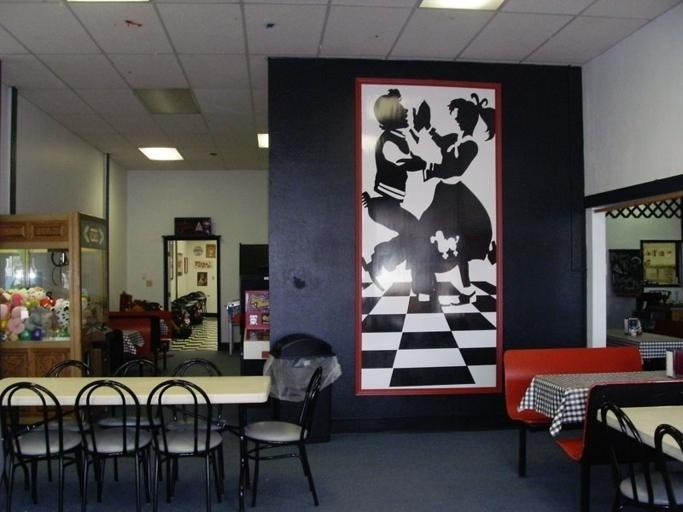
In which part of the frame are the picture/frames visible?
[355,78,503,396]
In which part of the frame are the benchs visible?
[504,347,642,478]
[553,382,682,511]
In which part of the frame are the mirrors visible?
[607,199,683,369]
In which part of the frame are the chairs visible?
[1,380,84,511]
[113,360,162,483]
[75,380,155,511]
[601,405,682,511]
[43,360,93,484]
[241,365,323,512]
[163,359,224,480]
[148,379,225,511]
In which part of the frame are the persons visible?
[361,89,439,303]
[395,92,497,308]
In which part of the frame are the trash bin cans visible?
[269,333,336,444]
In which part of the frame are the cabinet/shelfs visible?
[240,244,269,355]
[241,359,331,443]
[109,312,171,369]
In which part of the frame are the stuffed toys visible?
[0,286,104,343]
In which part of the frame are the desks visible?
[516,372,683,437]
[670,308,683,322]
[596,406,683,463]
[229,299,241,356]
[0,373,277,511]
[607,329,683,365]
